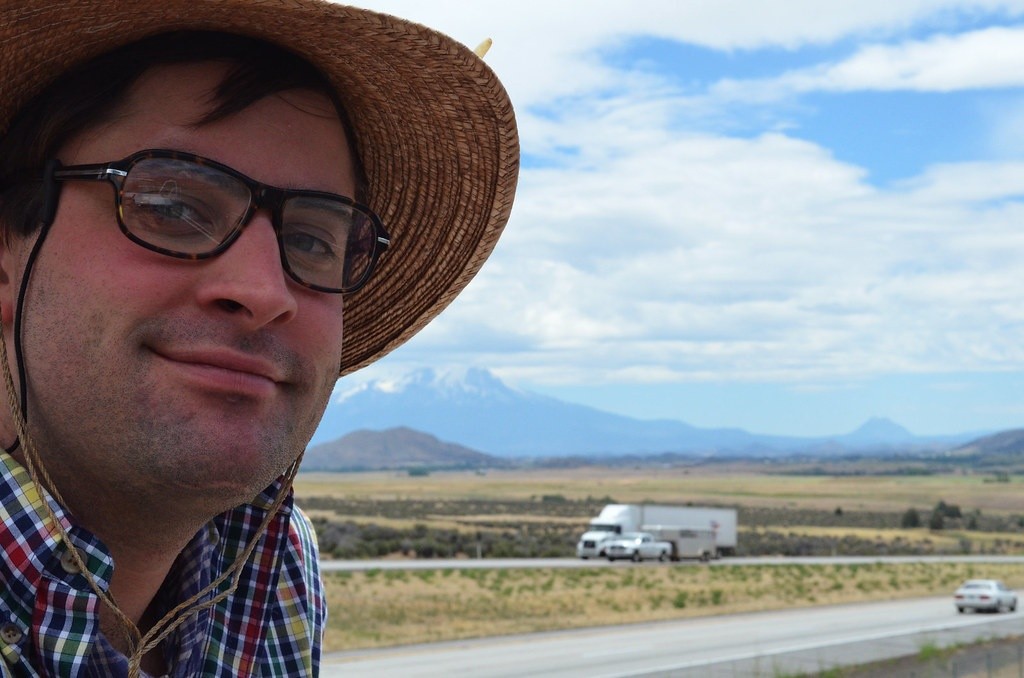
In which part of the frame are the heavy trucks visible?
[577,503,738,560]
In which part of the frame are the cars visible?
[953,578,1017,614]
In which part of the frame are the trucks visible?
[641,523,718,564]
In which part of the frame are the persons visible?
[0,0,520,678]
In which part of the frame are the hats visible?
[0,0,520,379]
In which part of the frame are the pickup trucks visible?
[605,529,674,563]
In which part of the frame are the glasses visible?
[19,149,387,294]
